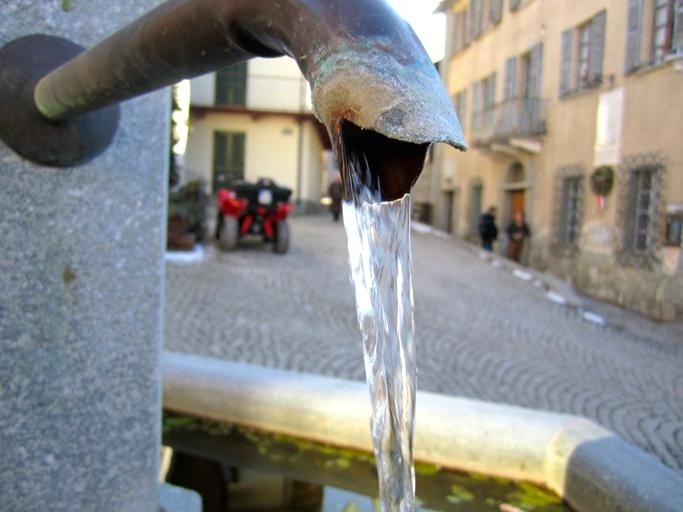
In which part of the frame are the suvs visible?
[215,179,293,254]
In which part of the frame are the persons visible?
[478,206,498,252]
[506,209,529,263]
[328,172,342,222]
